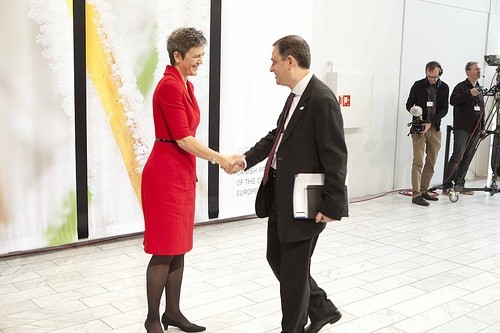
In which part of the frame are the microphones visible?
[410,106,423,116]
[480,75,485,77]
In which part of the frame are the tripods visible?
[446,92,500,195]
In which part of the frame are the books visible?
[292,173,350,219]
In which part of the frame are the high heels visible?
[161,312,206,332]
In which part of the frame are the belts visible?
[155,138,176,142]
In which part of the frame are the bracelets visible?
[211,152,219,164]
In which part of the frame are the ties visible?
[262,92,295,186]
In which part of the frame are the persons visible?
[406,61,449,206]
[224,36,349,333]
[140,28,244,333]
[442,61,485,195]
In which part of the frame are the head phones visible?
[428,62,443,76]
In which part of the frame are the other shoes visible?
[421,192,438,200]
[412,196,430,205]
[442,188,453,195]
[455,184,465,190]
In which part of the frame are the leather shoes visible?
[305,309,342,332]
